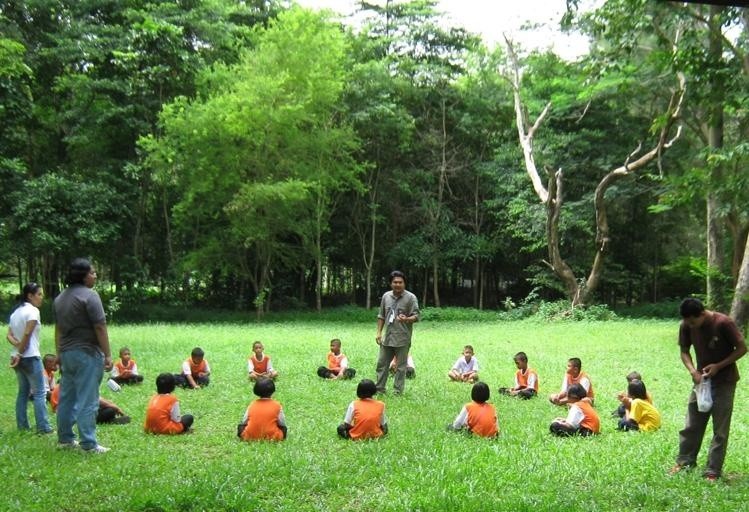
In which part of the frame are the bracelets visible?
[15,351,23,356]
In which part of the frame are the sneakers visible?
[113,416,131,424]
[45,429,57,435]
[57,440,80,448]
[81,445,111,453]
[702,472,720,481]
[672,463,697,473]
[392,390,403,396]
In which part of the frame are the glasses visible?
[30,283,37,292]
[87,272,96,276]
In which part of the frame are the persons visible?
[246,341,278,382]
[668,296,748,483]
[446,381,499,441]
[28,353,59,401]
[316,338,356,380]
[375,270,420,396]
[549,357,594,406]
[172,347,211,390]
[614,378,662,433]
[53,257,113,455]
[5,282,56,435]
[97,395,132,425]
[50,370,62,413]
[498,351,538,400]
[611,370,653,417]
[448,344,480,383]
[336,379,388,440]
[237,378,288,441]
[144,372,193,435]
[388,353,416,380]
[549,382,600,437]
[111,346,144,385]
